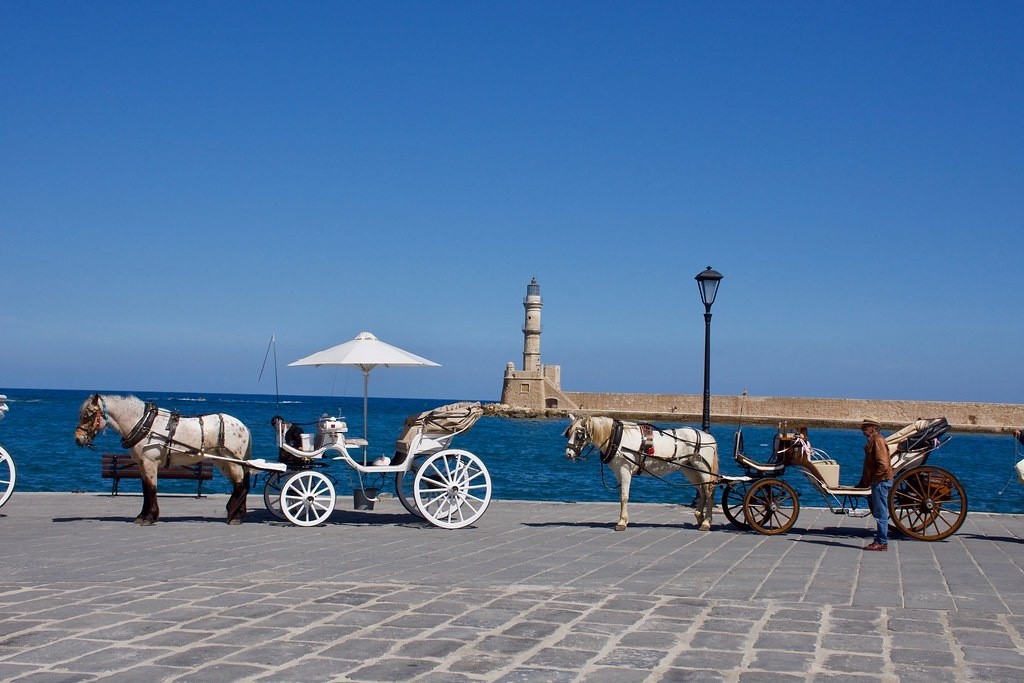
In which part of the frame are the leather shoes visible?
[864,542,888,551]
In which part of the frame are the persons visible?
[854,416,893,551]
[271,416,304,515]
[1013,429,1024,446]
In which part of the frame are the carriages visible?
[560,389,968,541]
[74,332,492,529]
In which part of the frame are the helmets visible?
[271,416,283,426]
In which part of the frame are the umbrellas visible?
[286,331,443,466]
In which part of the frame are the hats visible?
[859,418,882,426]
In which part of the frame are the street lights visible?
[691,266,724,507]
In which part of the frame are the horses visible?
[74,393,253,526]
[564,414,718,531]
